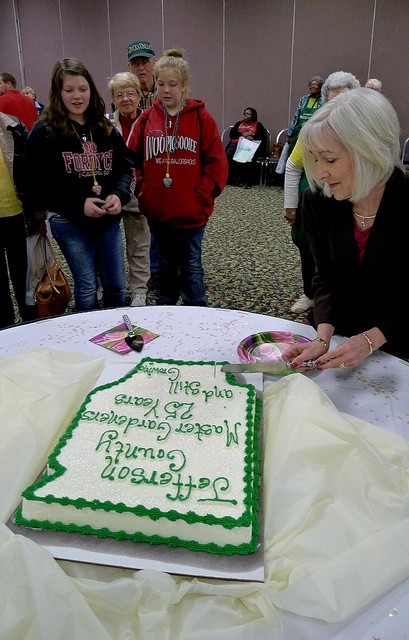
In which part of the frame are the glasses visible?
[112,91,138,99]
[129,59,151,68]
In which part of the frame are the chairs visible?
[399,137,409,172]
[265,128,291,188]
[221,125,271,186]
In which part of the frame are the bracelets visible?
[361,333,373,355]
[314,338,328,349]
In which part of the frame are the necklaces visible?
[353,211,376,226]
[162,104,183,153]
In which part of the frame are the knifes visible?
[221,359,324,373]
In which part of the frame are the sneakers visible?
[128,292,146,307]
[289,293,314,314]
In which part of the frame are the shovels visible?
[122,314,144,353]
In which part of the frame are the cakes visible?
[11,356,263,556]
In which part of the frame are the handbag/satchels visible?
[29,209,75,321]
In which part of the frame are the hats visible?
[126,40,154,62]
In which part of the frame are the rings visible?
[339,363,344,369]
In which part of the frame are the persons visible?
[104,71,152,307]
[21,86,45,115]
[125,48,229,306]
[0,72,39,133]
[225,107,267,189]
[126,39,159,112]
[17,58,134,310]
[0,111,47,328]
[284,71,363,313]
[286,76,324,156]
[281,87,409,372]
